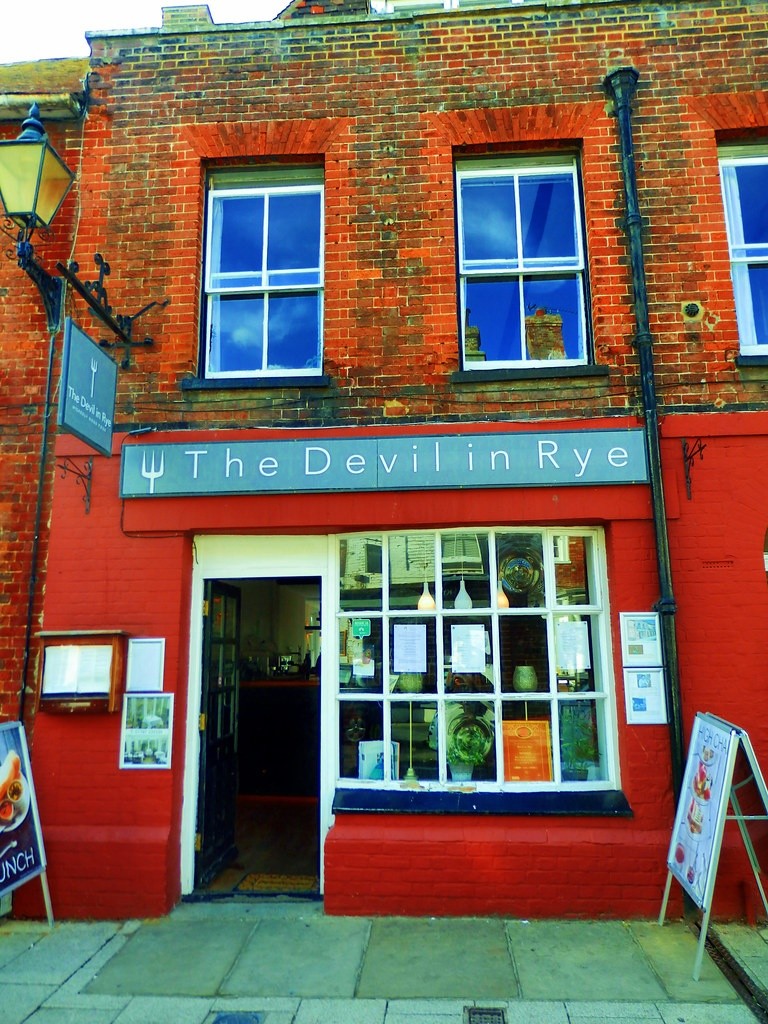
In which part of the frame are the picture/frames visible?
[623,668,667,725]
[126,638,166,692]
[619,611,663,667]
[119,692,172,770]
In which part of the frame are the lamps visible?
[454,533,473,610]
[0,101,79,332]
[416,534,438,612]
[494,533,510,609]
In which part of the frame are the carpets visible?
[233,871,320,892]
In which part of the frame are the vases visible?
[559,702,603,782]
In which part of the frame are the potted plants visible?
[446,726,489,782]
